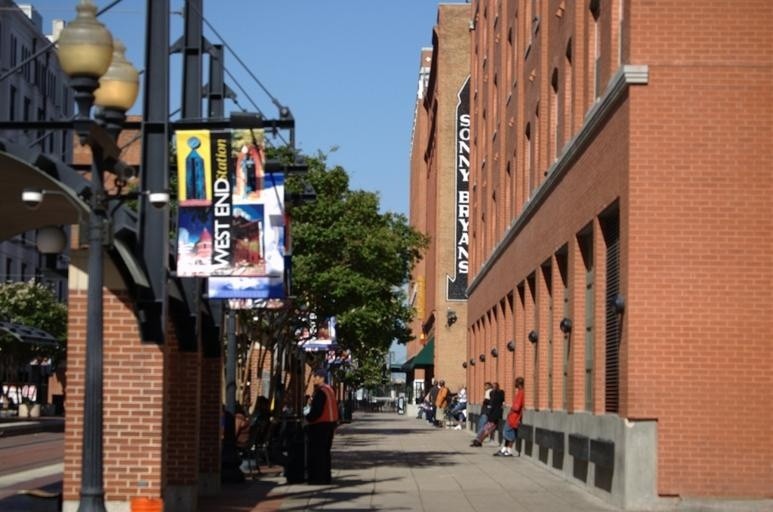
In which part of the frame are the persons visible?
[221,367,345,484]
[415,376,525,458]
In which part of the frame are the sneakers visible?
[471,439,513,456]
[416,408,468,430]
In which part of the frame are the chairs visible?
[235,422,263,481]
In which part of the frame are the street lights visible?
[20,1,169,512]
